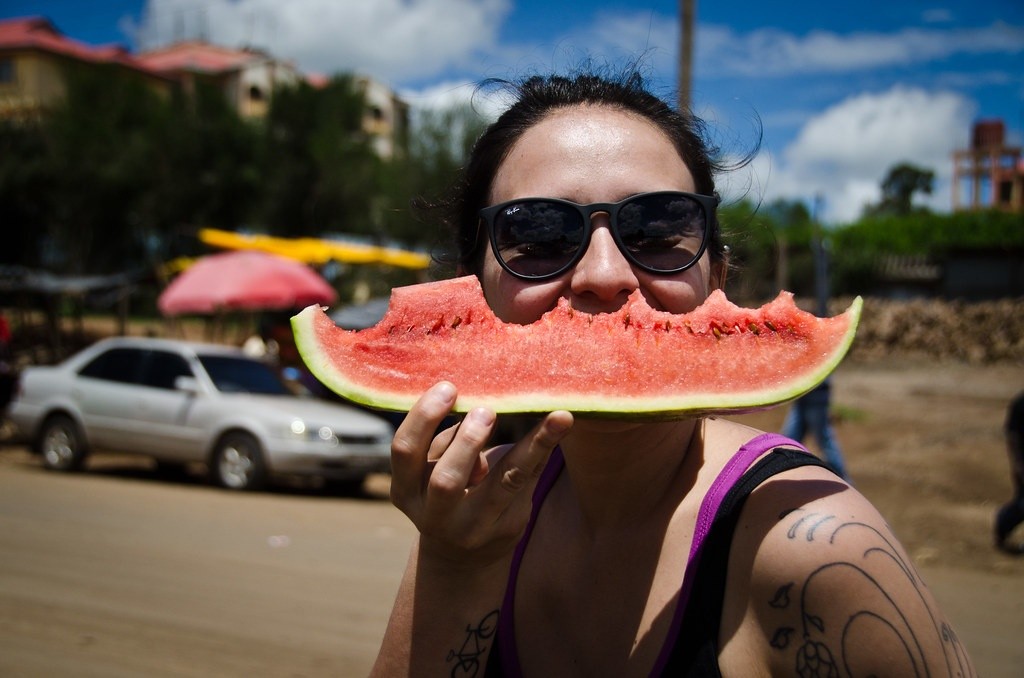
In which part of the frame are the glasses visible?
[476,190,718,281]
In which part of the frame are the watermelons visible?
[290,274,864,422]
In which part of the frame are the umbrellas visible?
[158,253,339,344]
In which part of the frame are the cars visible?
[7,333,397,502]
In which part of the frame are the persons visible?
[993,389,1024,558]
[779,379,849,486]
[365,57,978,678]
